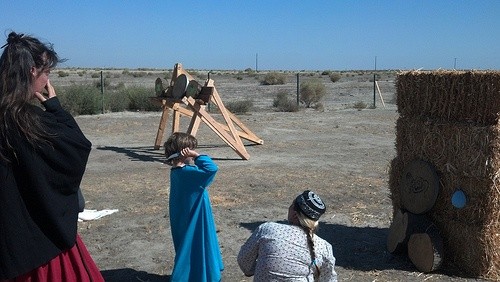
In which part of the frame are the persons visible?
[1,31,105,282]
[237,190,338,282]
[164,132,225,282]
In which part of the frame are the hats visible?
[294,190,325,222]
